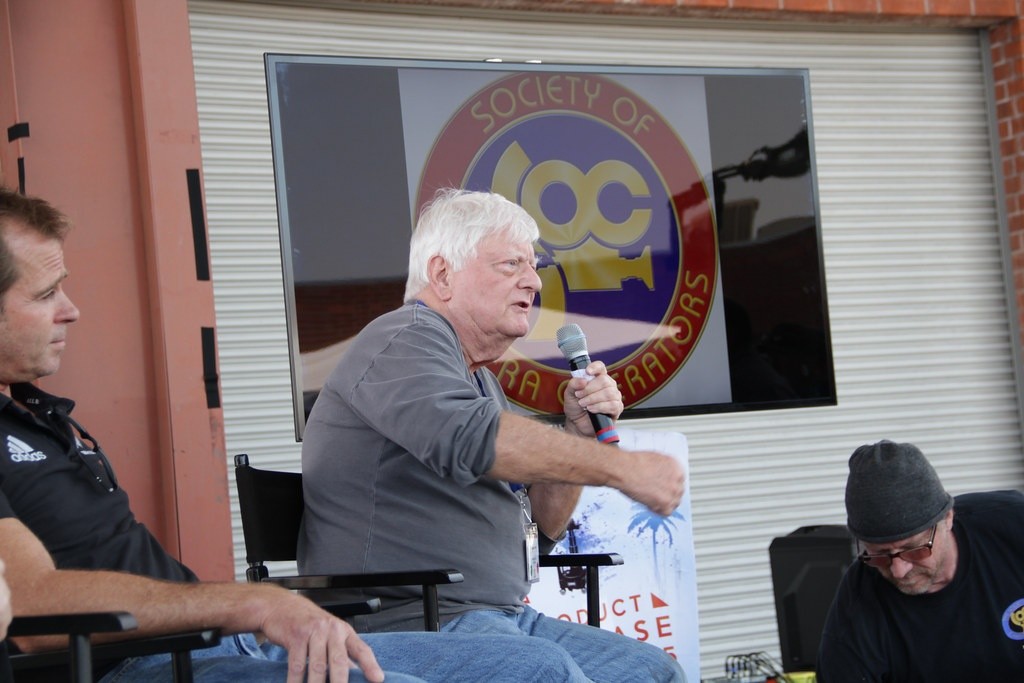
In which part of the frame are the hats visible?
[844,438,955,543]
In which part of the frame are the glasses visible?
[46,405,119,493]
[855,523,938,568]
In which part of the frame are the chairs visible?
[0,455,625,683]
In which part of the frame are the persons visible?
[814,437,1024,683]
[0,188,595,683]
[297,181,686,683]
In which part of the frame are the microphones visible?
[557,323,621,449]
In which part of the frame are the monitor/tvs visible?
[266,53,838,445]
